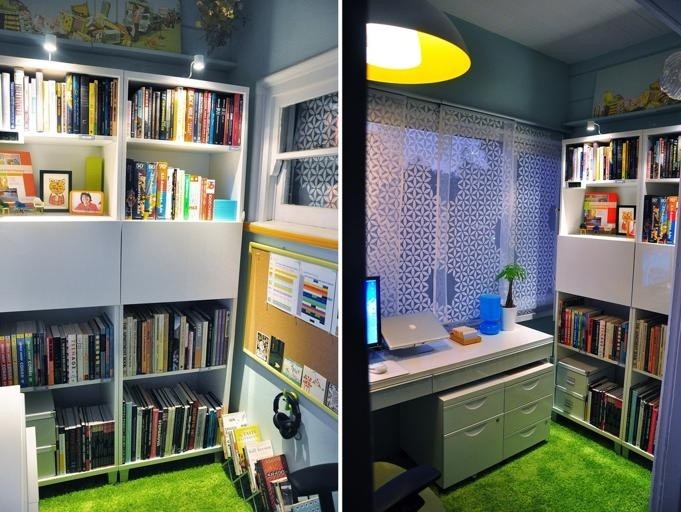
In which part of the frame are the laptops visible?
[381,310,450,351]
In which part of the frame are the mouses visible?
[368,361,387,374]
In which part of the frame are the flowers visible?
[195,0,246,53]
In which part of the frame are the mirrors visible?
[341,0,681,512]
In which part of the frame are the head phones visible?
[272,391,301,439]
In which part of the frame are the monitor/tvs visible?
[364,276,381,347]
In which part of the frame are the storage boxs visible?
[555,359,615,395]
[556,384,588,420]
[37,445,57,479]
[25,389,58,444]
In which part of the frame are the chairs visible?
[287,463,338,512]
[371,457,444,512]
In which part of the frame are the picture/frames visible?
[40,170,72,213]
[616,205,635,235]
[68,190,105,216]
[625,219,637,237]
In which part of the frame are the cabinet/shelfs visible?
[369,324,554,488]
[0,386,42,512]
[0,56,250,484]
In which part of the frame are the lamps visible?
[44,35,58,60]
[586,121,605,135]
[188,54,206,79]
[365,0,471,85]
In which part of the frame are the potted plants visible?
[494,264,526,332]
